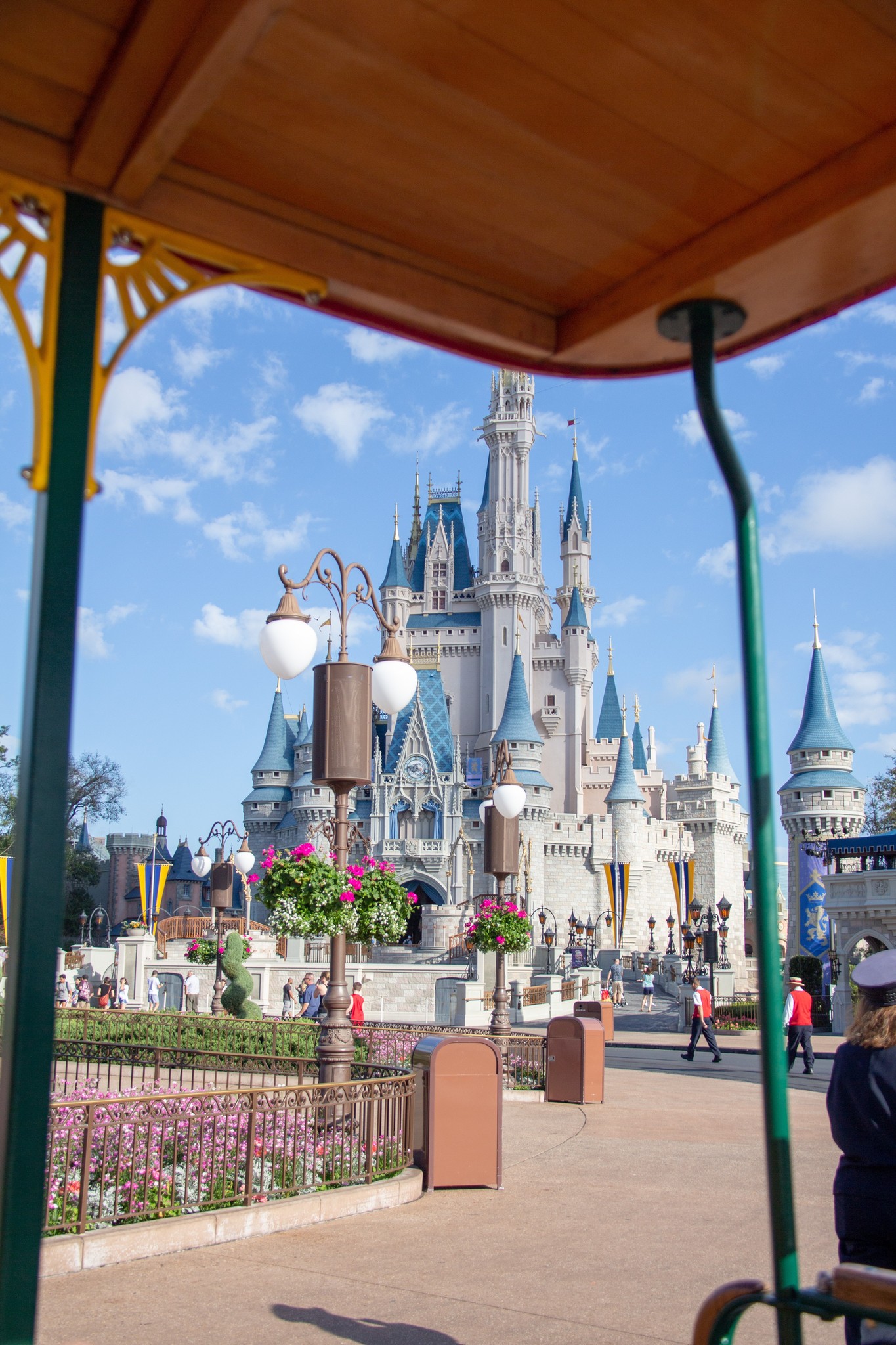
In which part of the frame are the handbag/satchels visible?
[298,991,303,998]
[99,993,109,1007]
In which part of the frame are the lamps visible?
[802,828,853,867]
[828,949,842,980]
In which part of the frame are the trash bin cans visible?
[544,1017,605,1104]
[407,1036,502,1189]
[573,1001,615,1040]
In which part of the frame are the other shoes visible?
[802,1068,814,1074]
[648,1010,651,1012]
[680,1053,695,1062]
[644,1003,647,1006]
[638,1009,643,1011]
[652,1002,656,1006]
[613,1004,616,1008]
[712,1056,722,1062]
[618,1004,621,1008]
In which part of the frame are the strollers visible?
[606,982,628,1007]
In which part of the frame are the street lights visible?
[462,932,478,981]
[267,548,418,1135]
[466,741,528,1046]
[78,903,112,949]
[647,913,657,951]
[538,906,556,975]
[192,820,255,1018]
[567,906,596,969]
[665,907,678,954]
[680,891,733,985]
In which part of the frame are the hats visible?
[408,935,411,938]
[786,977,805,987]
[850,948,896,1007]
[643,964,648,969]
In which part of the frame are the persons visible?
[295,972,320,1021]
[184,970,200,1015]
[606,959,623,1008]
[783,977,814,1074]
[118,978,129,1010]
[637,965,656,1006]
[314,971,330,1023]
[282,978,297,1019]
[71,977,82,1007]
[639,966,655,1012]
[97,977,114,1013]
[55,973,72,1008]
[221,979,228,992]
[681,977,722,1062]
[295,978,307,1007]
[825,948,896,1345]
[147,970,163,1011]
[346,982,364,1030]
[76,974,94,1009]
[600,984,613,1003]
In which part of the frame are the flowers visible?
[247,843,420,948]
[464,899,533,955]
[185,934,256,964]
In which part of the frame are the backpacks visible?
[78,980,90,999]
[405,939,410,944]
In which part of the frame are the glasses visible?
[304,978,311,980]
[320,976,325,978]
[690,983,693,987]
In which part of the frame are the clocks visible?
[402,752,431,783]
